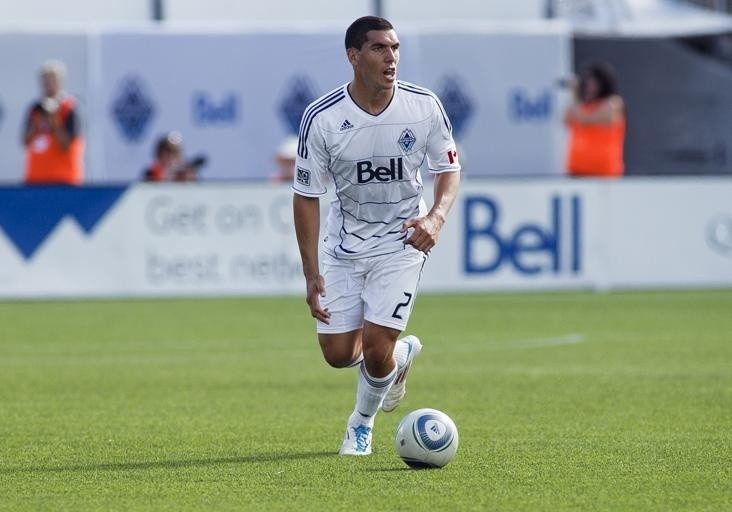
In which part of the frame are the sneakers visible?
[382,335,422,413]
[338,424,373,455]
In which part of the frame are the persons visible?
[291,15,461,456]
[143,139,205,182]
[561,65,626,175]
[21,60,85,187]
[272,136,299,179]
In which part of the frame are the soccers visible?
[394,408,459,467]
